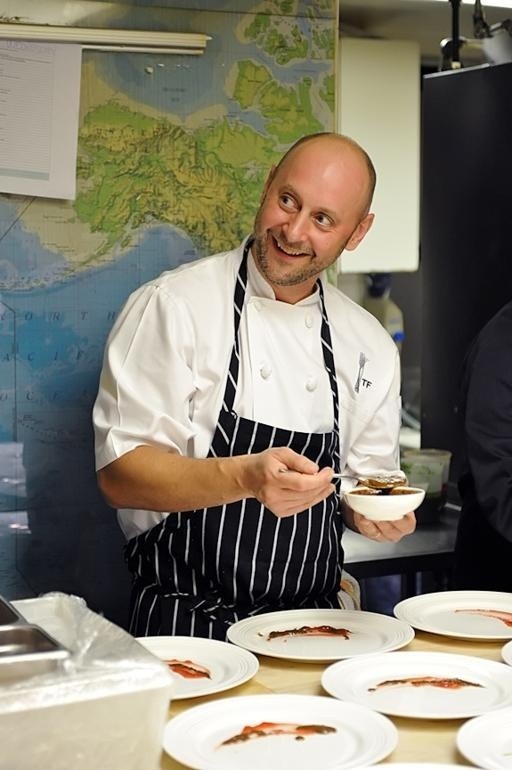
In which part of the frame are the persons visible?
[449,299,512,590]
[91,133,418,645]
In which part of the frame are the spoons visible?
[278,467,406,490]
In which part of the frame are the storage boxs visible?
[0,596,176,770]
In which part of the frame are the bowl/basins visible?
[343,486,426,522]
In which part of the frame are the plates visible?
[161,692,400,769]
[228,607,417,663]
[392,589,512,642]
[455,705,512,770]
[133,636,260,703]
[321,651,512,720]
[499,639,512,667]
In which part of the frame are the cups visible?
[404,448,451,490]
[400,457,444,504]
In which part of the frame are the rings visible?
[370,532,381,539]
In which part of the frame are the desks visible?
[338,504,467,603]
[156,623,511,770]
